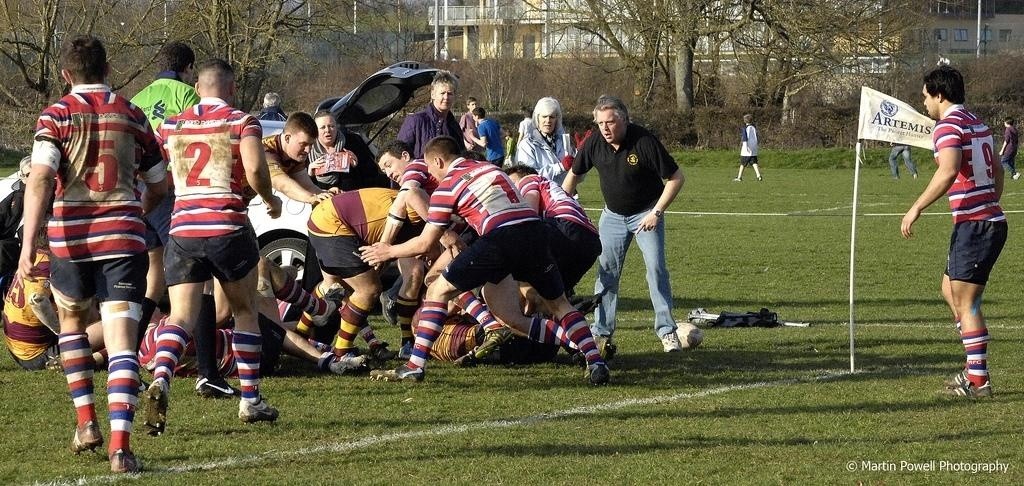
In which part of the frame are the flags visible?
[858,85,936,151]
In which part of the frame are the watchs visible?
[652,207,663,217]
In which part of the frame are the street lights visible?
[876,5,884,71]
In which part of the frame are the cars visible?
[0,60,460,289]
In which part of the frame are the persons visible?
[889,142,918,180]
[998,116,1022,180]
[561,94,682,352]
[900,65,1008,398]
[733,115,762,182]
[0,34,611,472]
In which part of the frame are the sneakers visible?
[71,421,103,453]
[108,448,139,473]
[569,335,614,387]
[945,367,992,401]
[311,283,426,382]
[474,325,512,357]
[195,374,242,398]
[661,331,682,352]
[238,395,278,423]
[143,379,168,437]
